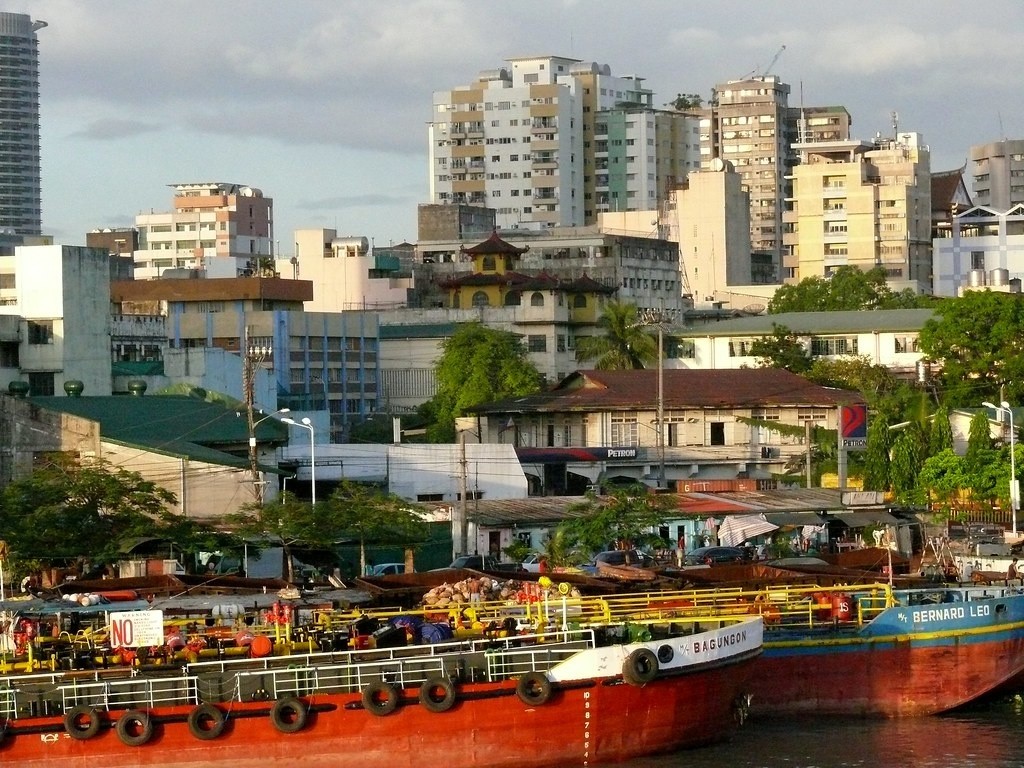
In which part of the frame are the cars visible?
[683,546,743,566]
[521,553,549,573]
[752,543,799,562]
[370,563,418,575]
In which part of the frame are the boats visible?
[746,581,1024,719]
[1,575,753,760]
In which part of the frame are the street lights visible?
[247,408,292,518]
[281,417,318,527]
[631,296,682,487]
[982,400,1016,535]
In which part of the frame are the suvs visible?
[576,549,645,576]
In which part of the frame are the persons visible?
[491,541,498,557]
[539,557,547,572]
[706,556,712,563]
[197,560,205,574]
[619,538,628,549]
[679,536,684,548]
[238,566,245,577]
[29,571,38,588]
[1006,558,1022,580]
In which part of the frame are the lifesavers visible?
[115,709,154,746]
[516,671,552,707]
[187,703,225,740]
[64,704,100,740]
[361,681,398,716]
[270,696,307,733]
[622,648,658,686]
[419,677,456,713]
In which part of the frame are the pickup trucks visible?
[428,555,523,574]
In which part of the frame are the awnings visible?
[836,512,897,527]
[766,512,824,525]
